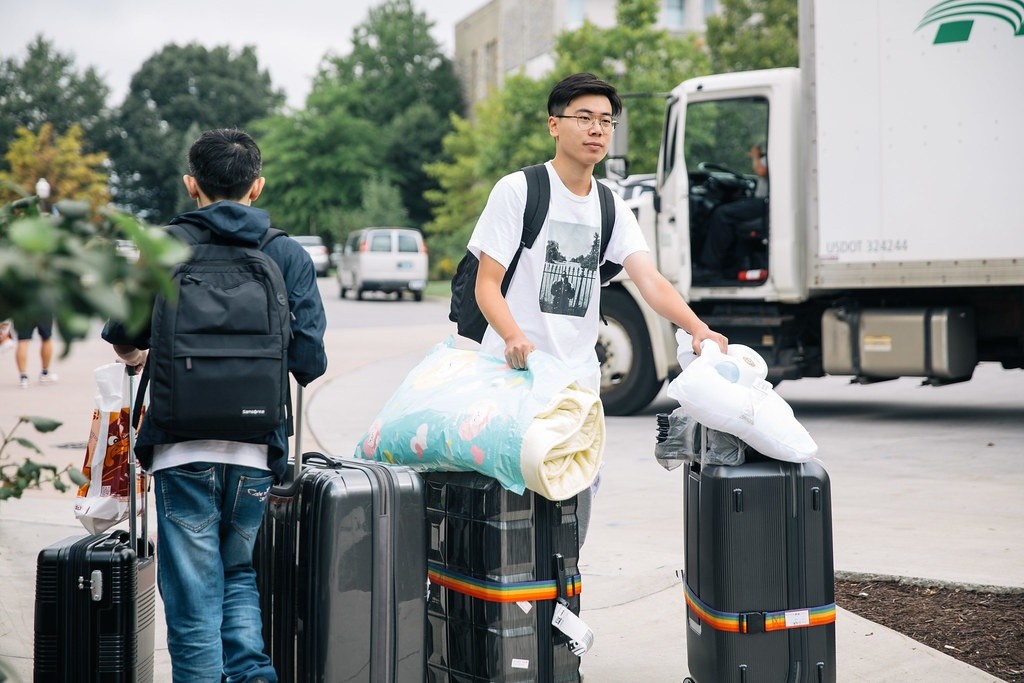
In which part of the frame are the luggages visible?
[251,383,425,683]
[419,472,594,683]
[684,421,836,683]
[33,363,155,683]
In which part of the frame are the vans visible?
[335,227,429,303]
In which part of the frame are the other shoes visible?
[21,375,27,387]
[41,371,59,383]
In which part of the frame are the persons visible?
[100,130,327,683]
[465,71,729,551]
[695,141,767,272]
[15,309,60,388]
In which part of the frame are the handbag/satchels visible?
[666,329,818,464]
[73,364,150,535]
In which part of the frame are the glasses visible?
[555,114,618,134]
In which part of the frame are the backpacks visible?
[448,163,615,344]
[151,224,291,436]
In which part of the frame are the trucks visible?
[595,0,1023,416]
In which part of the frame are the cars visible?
[286,235,330,277]
